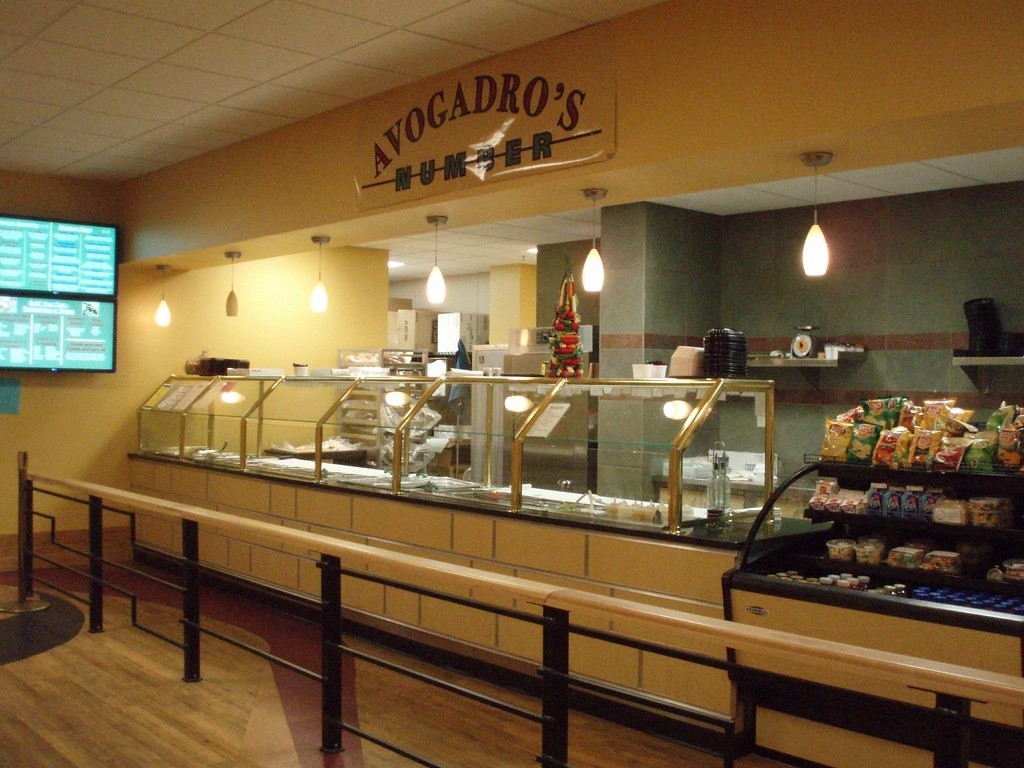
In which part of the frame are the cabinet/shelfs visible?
[718,396,1023,766]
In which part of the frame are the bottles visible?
[707,460,732,522]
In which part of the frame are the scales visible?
[788,323,821,359]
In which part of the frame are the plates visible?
[701,328,748,380]
[952,296,1024,357]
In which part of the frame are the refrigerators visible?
[501,325,599,497]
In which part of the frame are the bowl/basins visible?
[631,363,651,380]
[650,365,668,379]
[293,367,309,378]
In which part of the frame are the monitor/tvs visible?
[0,213,119,373]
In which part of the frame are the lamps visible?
[423,215,449,304]
[153,264,169,324]
[797,149,833,280]
[580,187,607,295]
[309,233,332,312]
[222,249,244,319]
